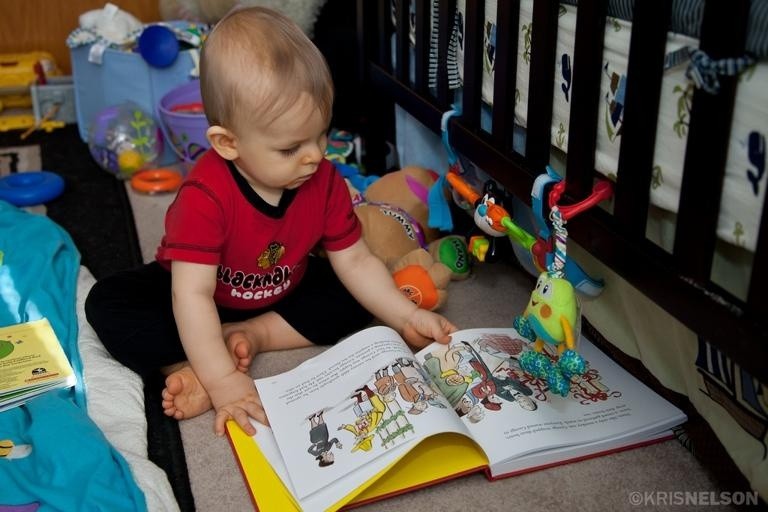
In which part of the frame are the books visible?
[1,317,75,411]
[220,324,689,512]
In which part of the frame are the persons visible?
[84,4,456,437]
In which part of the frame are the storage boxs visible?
[70,46,201,166]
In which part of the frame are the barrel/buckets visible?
[155,81,212,172]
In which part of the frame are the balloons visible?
[140,24,180,68]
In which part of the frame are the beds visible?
[344,0,768,391]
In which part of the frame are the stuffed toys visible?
[354,167,472,312]
[333,163,380,205]
[512,273,585,395]
